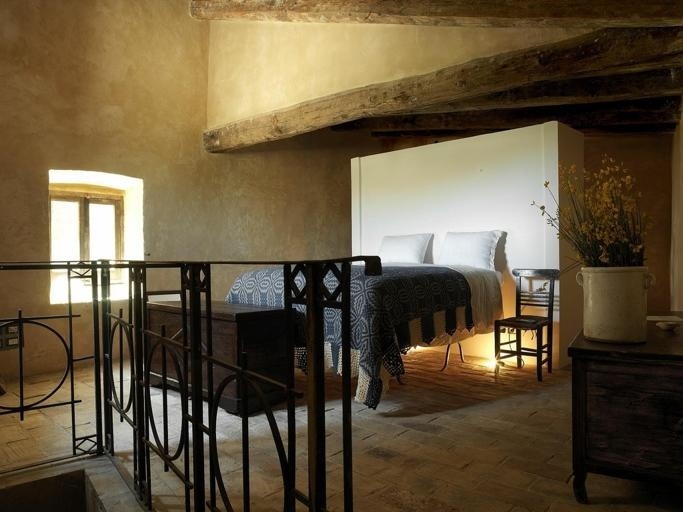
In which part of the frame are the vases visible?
[568,261,656,345]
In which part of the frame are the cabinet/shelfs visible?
[564,309,682,512]
[134,297,305,418]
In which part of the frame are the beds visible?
[219,258,514,413]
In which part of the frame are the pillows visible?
[428,228,505,272]
[374,231,434,267]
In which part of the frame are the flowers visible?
[523,144,659,270]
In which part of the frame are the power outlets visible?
[507,327,516,334]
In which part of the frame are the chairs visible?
[492,266,561,384]
